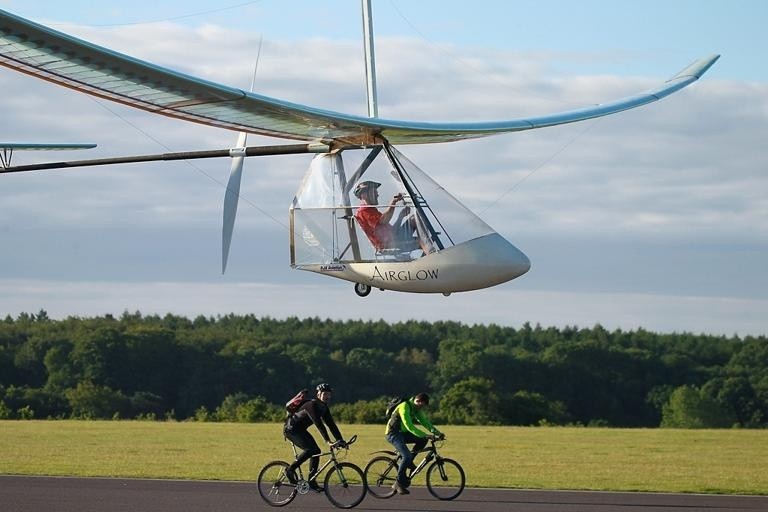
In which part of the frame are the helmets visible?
[353,180,382,200]
[316,383,335,392]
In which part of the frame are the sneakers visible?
[281,467,298,486]
[407,462,417,471]
[390,480,411,496]
[308,482,326,494]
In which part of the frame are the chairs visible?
[351,215,412,263]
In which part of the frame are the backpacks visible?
[385,394,413,419]
[284,388,309,414]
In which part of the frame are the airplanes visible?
[0,0,722,297]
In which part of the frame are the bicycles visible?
[258,433,466,509]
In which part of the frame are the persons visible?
[353,180,430,256]
[384,391,445,494]
[283,383,349,493]
